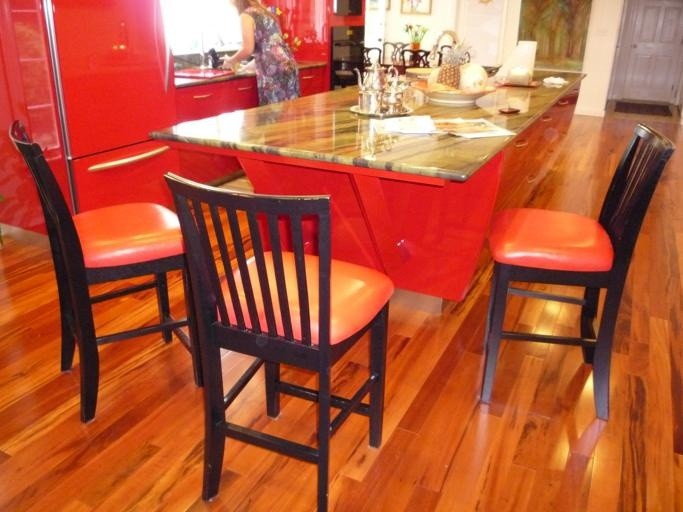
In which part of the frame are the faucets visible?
[199,29,227,66]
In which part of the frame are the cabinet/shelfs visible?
[176,67,324,188]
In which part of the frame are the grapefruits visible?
[458,63,488,93]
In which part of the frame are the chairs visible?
[164,172,395,512]
[8,119,205,424]
[333,30,470,89]
[480,123,676,422]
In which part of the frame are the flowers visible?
[405,25,428,42]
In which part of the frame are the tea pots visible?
[353,62,399,91]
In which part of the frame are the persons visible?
[221,0,301,104]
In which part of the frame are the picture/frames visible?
[400,0,432,16]
[369,0,391,11]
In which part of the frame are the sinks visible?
[201,60,252,76]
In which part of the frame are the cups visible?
[358,76,407,114]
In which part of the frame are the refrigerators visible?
[1,2,183,241]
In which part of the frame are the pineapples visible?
[436,38,473,90]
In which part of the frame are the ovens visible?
[331,27,364,89]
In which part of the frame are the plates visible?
[349,105,414,117]
[410,81,497,104]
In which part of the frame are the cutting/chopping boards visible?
[173,68,235,80]
